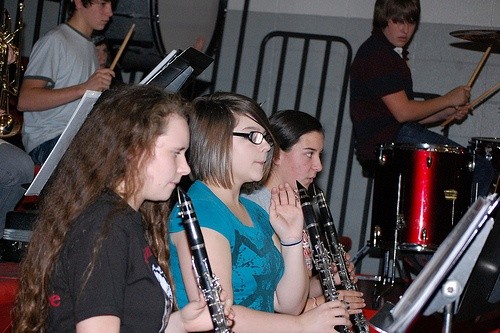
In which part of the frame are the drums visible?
[355,274,421,333]
[369,142,468,251]
[464,136,500,238]
[63,0,228,72]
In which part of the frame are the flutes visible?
[176,182,235,333]
[296,180,349,333]
[313,179,369,333]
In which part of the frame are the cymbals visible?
[450,30,500,43]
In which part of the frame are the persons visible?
[16,0,115,164]
[240,109,366,315]
[0,42,35,261]
[11,84,236,333]
[167,91,353,333]
[349,1,496,268]
[90,34,124,88]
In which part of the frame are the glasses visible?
[231,131,274,147]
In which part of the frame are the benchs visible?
[0,262,22,333]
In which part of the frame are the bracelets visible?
[311,296,318,309]
[280,239,303,247]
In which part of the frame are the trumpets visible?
[0,8,21,137]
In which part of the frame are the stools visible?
[352,167,411,308]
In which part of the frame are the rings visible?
[346,302,350,310]
